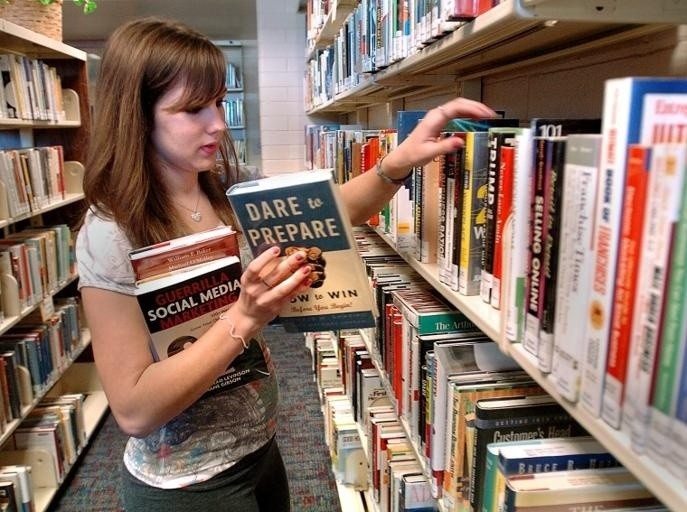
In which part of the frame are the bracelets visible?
[377,156,414,184]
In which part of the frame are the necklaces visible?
[172,188,203,222]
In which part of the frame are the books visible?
[395,110,603,414]
[303,0,386,111]
[219,139,244,163]
[226,169,378,335]
[434,341,666,512]
[587,76,685,490]
[305,0,337,55]
[306,330,391,407]
[355,226,493,463]
[0,53,66,123]
[306,124,397,242]
[0,218,76,322]
[0,297,81,434]
[332,407,438,512]
[224,63,240,88]
[0,394,86,512]
[221,98,243,128]
[386,1,496,68]
[136,256,270,395]
[131,227,240,278]
[1,128,66,220]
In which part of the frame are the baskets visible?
[1,1,63,40]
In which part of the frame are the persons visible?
[75,17,502,512]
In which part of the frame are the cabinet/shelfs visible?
[0,18,111,512]
[300,2,686,511]
[220,46,247,178]
